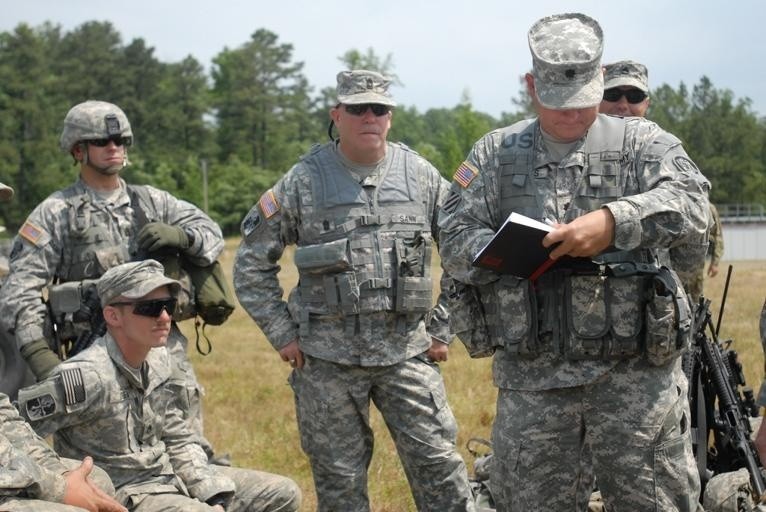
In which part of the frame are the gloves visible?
[131,220,192,255]
[18,338,61,380]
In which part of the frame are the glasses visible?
[603,86,649,104]
[111,297,180,317]
[89,136,131,147]
[342,104,390,116]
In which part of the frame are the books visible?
[469,209,602,288]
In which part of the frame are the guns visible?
[688,258,766,500]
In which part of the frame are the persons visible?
[434,10,712,512]
[0,390,136,512]
[0,92,228,385]
[0,256,306,512]
[230,68,477,512]
[599,59,652,117]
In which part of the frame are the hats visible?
[527,10,606,111]
[95,258,182,306]
[603,58,650,95]
[335,71,396,107]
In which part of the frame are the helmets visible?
[58,100,133,150]
